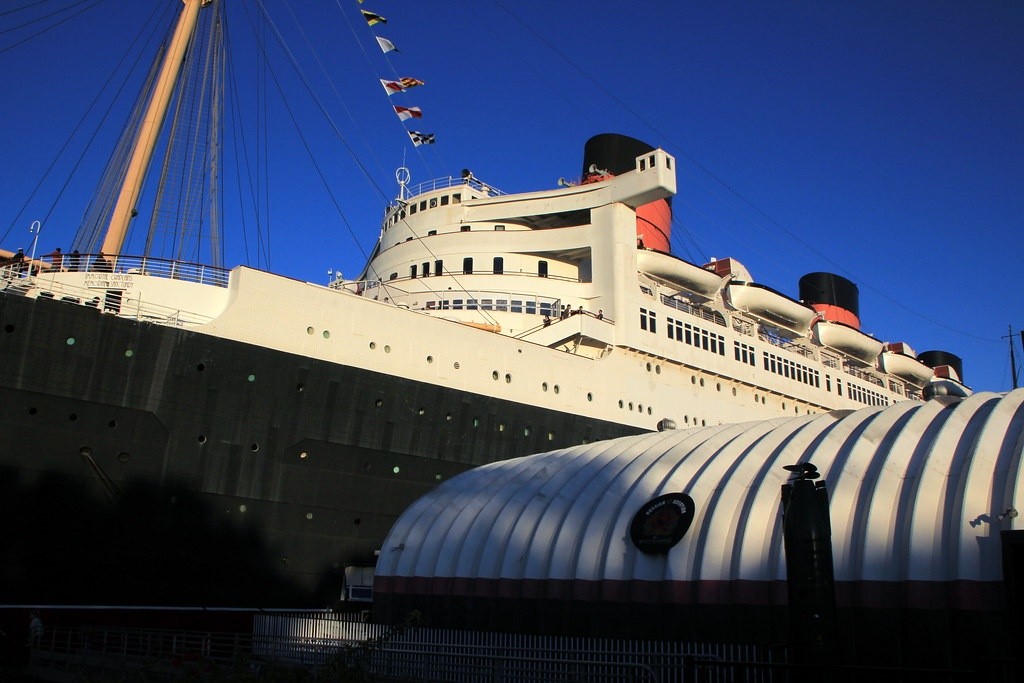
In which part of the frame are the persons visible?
[597,308,603,320]
[9,247,125,278]
[560,304,572,319]
[577,305,585,314]
[543,315,552,328]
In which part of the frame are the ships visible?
[0,0,975,613]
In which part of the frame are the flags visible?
[392,103,423,122]
[407,129,436,148]
[360,9,388,27]
[374,35,402,57]
[379,78,407,96]
[399,76,425,90]
